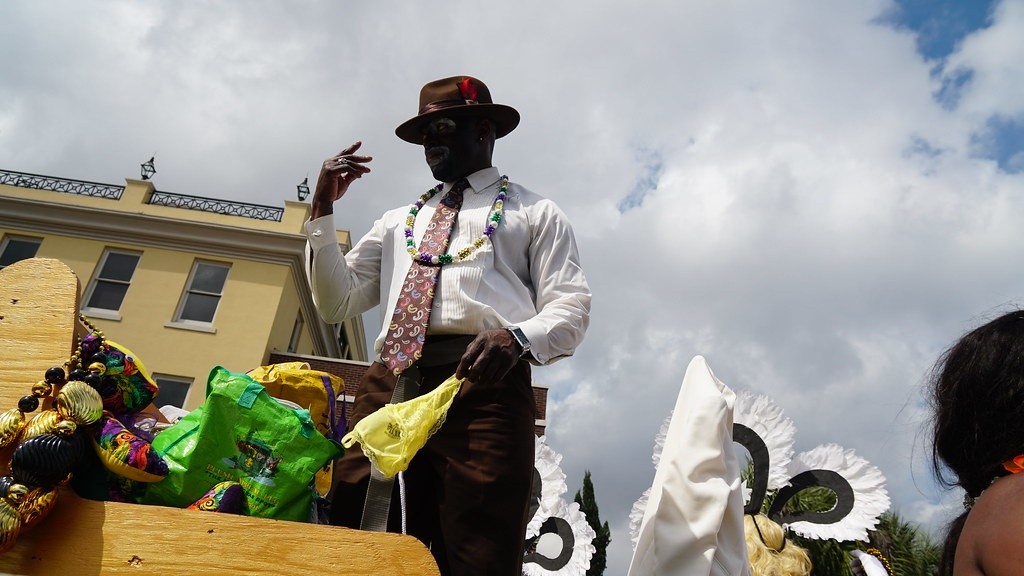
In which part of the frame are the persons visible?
[743,511,896,576]
[931,310,1024,576]
[304,75,591,576]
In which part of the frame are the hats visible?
[394,76,520,145]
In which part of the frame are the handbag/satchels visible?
[245,362,347,442]
[144,365,346,521]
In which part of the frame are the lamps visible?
[139,156,157,180]
[296,175,310,201]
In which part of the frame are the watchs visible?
[506,325,531,358]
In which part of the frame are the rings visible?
[337,158,347,165]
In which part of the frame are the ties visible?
[379,178,472,376]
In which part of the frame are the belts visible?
[360,334,477,533]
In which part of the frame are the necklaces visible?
[405,174,508,264]
